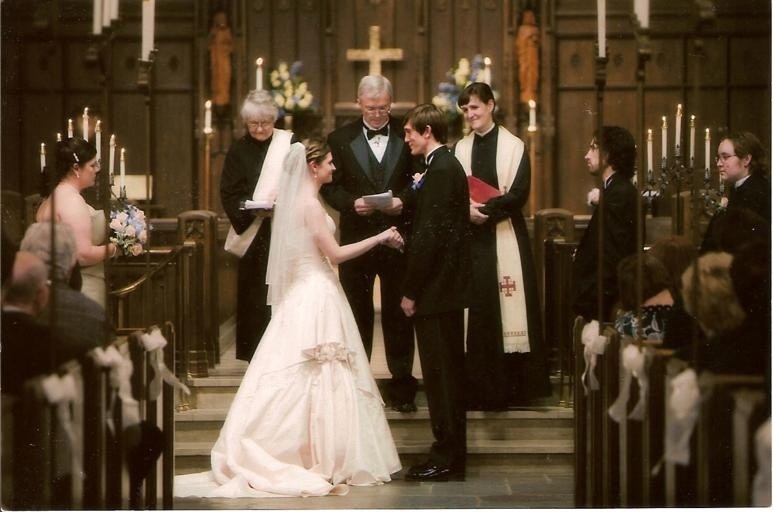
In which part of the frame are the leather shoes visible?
[391,399,416,414]
[406,460,468,483]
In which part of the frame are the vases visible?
[283,115,292,129]
[462,113,470,138]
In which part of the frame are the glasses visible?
[244,120,273,130]
[360,99,392,115]
[714,153,739,163]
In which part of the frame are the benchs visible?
[2,322,173,509]
[573,315,771,507]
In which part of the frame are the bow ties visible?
[365,127,389,141]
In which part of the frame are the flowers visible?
[432,54,499,115]
[410,172,423,190]
[269,60,312,113]
[108,205,154,257]
[587,188,599,206]
[718,197,728,209]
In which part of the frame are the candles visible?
[204,101,211,129]
[38,106,125,188]
[93,2,155,61]
[528,99,536,127]
[595,0,648,57]
[256,57,263,90]
[647,103,725,192]
[484,58,491,85]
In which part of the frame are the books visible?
[362,190,393,211]
[244,200,274,210]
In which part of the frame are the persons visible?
[385,103,473,483]
[571,125,645,323]
[0,138,129,399]
[219,90,300,365]
[612,232,771,377]
[516,10,540,104]
[319,74,426,415]
[207,11,234,105]
[697,131,770,255]
[210,132,406,498]
[451,82,553,412]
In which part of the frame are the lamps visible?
[111,176,152,206]
